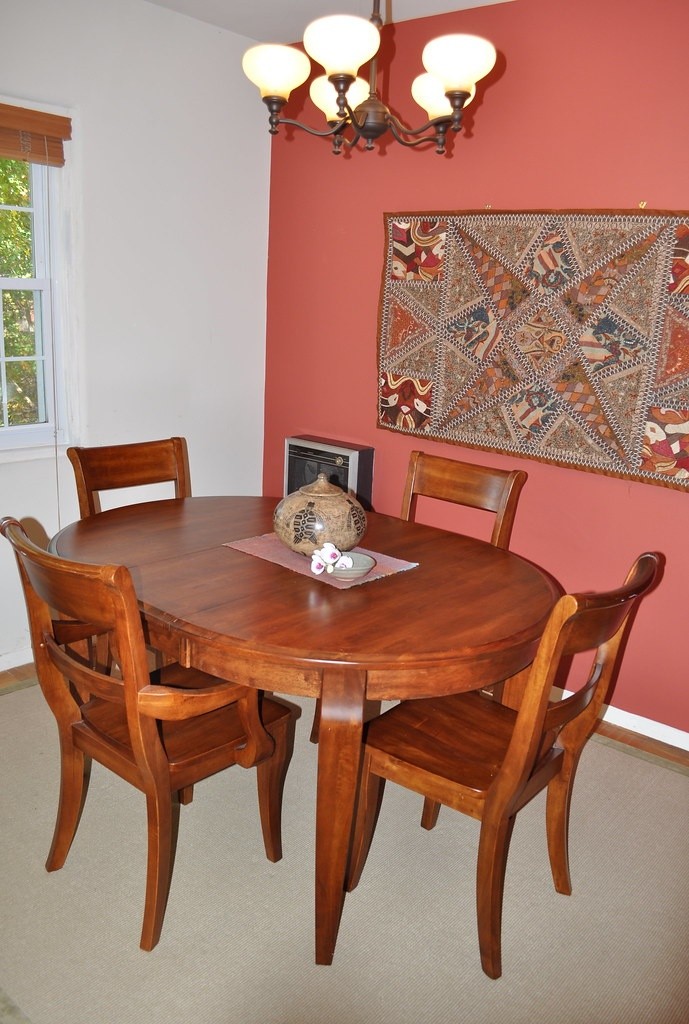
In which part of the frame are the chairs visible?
[0,515,303,954]
[65,433,200,673]
[308,446,531,744]
[347,548,668,985]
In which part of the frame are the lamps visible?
[242,0,504,158]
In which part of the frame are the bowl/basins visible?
[326,552,376,582]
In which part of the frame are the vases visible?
[325,553,377,582]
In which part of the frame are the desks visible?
[44,494,572,964]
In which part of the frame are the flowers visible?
[307,541,356,577]
